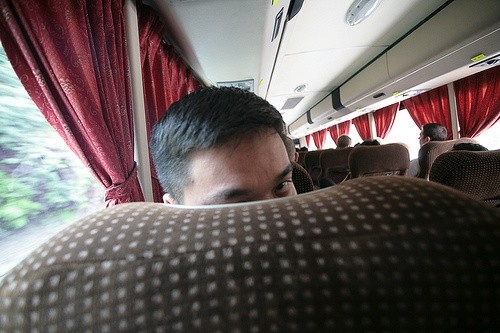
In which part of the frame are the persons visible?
[149,86,298,206]
[406,123,448,178]
[281,135,299,164]
[451,141,488,153]
[336,134,352,149]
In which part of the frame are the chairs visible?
[0,137,500,333]
